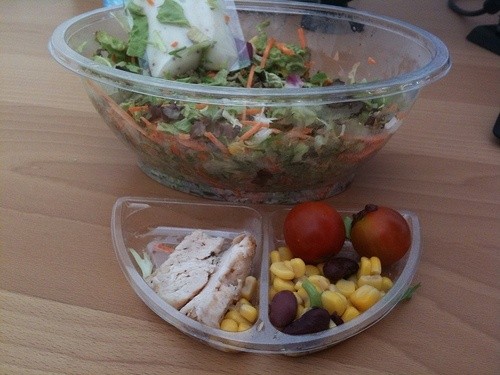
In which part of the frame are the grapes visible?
[270,257,359,335]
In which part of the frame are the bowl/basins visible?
[42,0,452,207]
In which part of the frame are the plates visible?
[110,196,420,358]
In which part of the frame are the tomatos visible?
[283,202,412,265]
